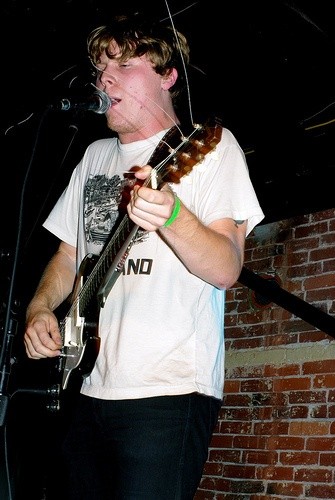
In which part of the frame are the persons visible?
[21,13,265,500]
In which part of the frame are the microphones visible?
[40,90,112,114]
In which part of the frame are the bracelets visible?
[160,192,181,232]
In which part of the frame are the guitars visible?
[44,114,224,412]
